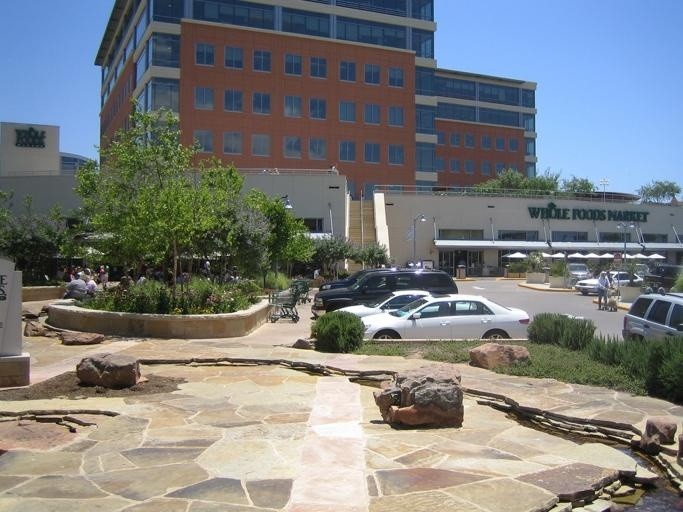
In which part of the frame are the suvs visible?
[567,262,592,285]
[645,265,683,295]
[621,294,683,346]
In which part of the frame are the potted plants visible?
[616,262,641,302]
[504,250,570,288]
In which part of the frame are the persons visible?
[597,270,610,310]
[584,268,614,298]
[54,260,240,299]
[366,261,402,271]
[312,266,324,282]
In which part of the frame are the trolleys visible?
[269,279,314,323]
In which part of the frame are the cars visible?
[575,271,644,295]
[312,268,530,340]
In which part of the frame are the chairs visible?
[437,304,450,316]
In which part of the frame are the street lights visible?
[617,222,635,264]
[267,194,292,227]
[413,213,426,265]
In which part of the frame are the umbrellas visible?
[500,250,513,263]
[507,251,666,263]
